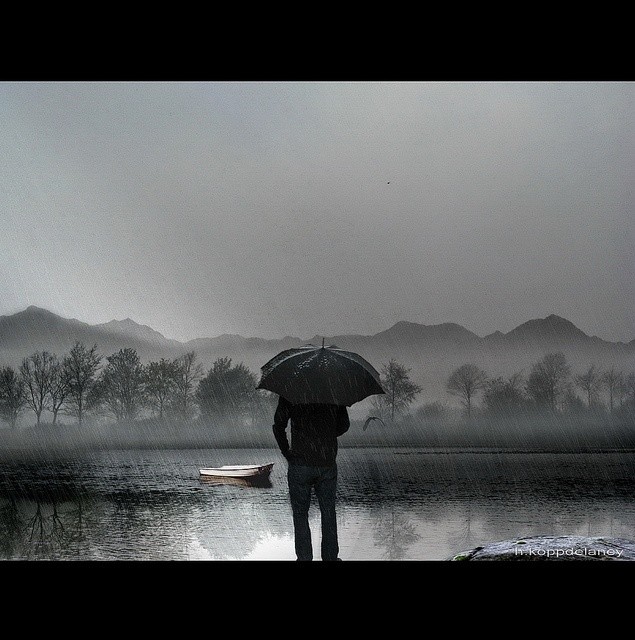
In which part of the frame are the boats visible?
[198,462,274,478]
[199,476,273,489]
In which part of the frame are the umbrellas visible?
[253,336,386,406]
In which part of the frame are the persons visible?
[273,394,351,561]
